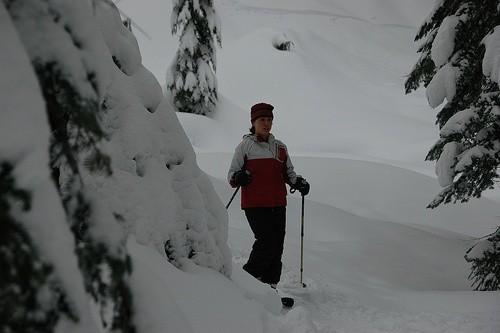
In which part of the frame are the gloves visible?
[293,177,310,196]
[232,170,252,186]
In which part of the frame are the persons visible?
[226,102,310,292]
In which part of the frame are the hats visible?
[250,103,274,123]
